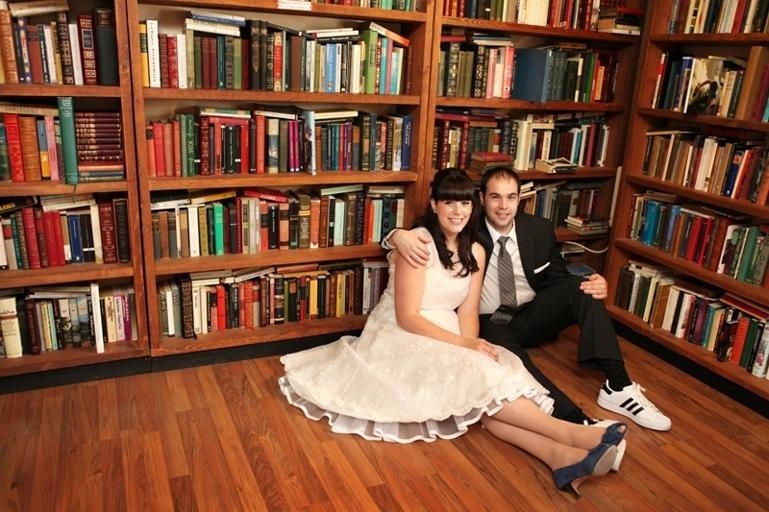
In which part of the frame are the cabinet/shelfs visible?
[604,0,768,400]
[1,2,641,385]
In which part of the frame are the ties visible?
[489,237,518,326]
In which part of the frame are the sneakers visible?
[584,412,624,471]
[597,378,672,432]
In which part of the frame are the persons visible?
[380,165,672,475]
[277,169,628,501]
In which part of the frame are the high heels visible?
[552,423,628,499]
